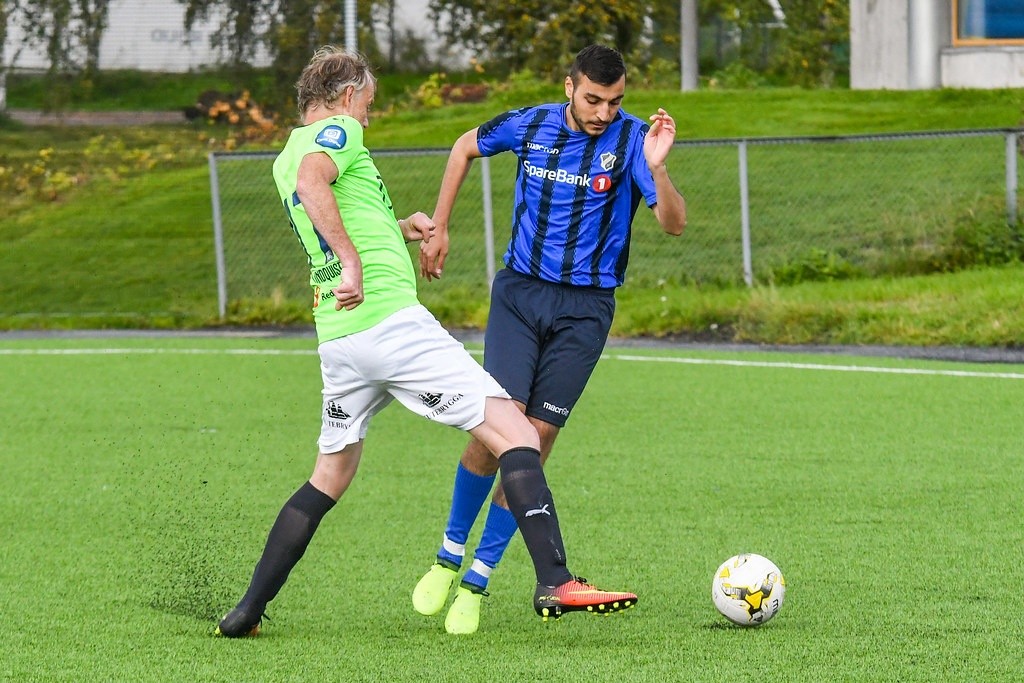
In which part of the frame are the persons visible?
[413,47,687,634]
[213,46,637,641]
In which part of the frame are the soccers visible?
[713,553,785,627]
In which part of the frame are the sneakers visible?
[444,589,486,635]
[217,611,262,637]
[535,579,636,623]
[411,560,458,617]
